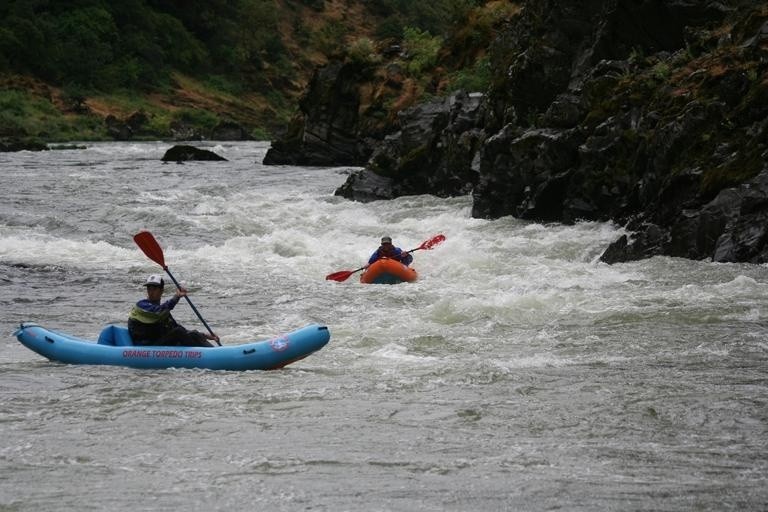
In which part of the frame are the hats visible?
[381,236,392,244]
[143,274,164,289]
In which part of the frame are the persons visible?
[365,236,413,268]
[129,275,220,347]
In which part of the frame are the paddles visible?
[134,231,222,348]
[325,235,446,282]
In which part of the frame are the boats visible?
[360,258,421,285]
[15,318,331,371]
